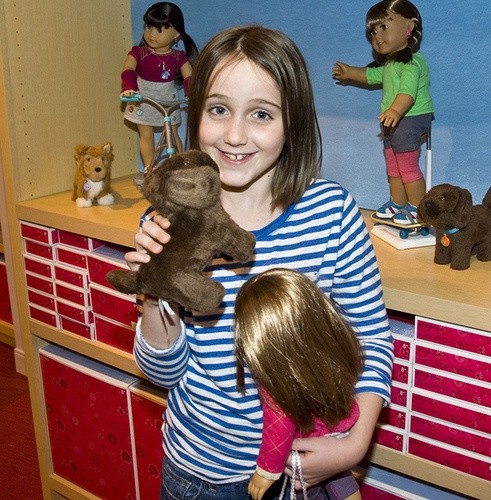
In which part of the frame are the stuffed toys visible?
[70,140,119,208]
[418,184,491,271]
[107,150,256,315]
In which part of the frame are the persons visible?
[331,0,435,226]
[232,269,364,500]
[124,26,394,500]
[121,2,198,177]
